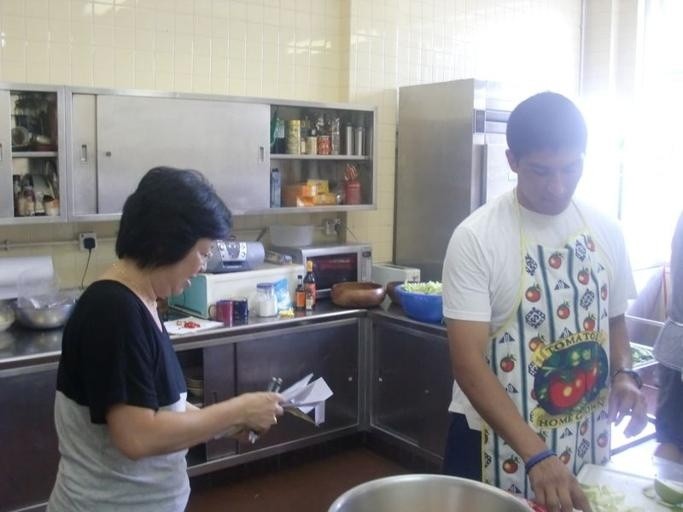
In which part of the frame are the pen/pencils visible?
[252,377,283,446]
[247,375,277,441]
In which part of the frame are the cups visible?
[208,300,234,329]
[346,180,361,205]
[232,299,249,326]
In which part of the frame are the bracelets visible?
[524,450,557,474]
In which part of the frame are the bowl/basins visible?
[327,473,534,512]
[15,296,73,329]
[387,280,416,304]
[394,282,444,324]
[331,280,386,308]
[185,387,205,397]
[185,378,204,387]
[269,225,313,248]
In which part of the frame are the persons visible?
[653,207,683,464]
[46,166,286,511]
[441,91,647,512]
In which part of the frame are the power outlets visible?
[324,218,340,235]
[78,232,96,252]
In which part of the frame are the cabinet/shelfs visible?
[0,298,454,512]
[393,79,518,281]
[0,83,379,227]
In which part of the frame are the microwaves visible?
[268,243,373,300]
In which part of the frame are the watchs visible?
[613,368,643,389]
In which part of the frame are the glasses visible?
[188,245,220,270]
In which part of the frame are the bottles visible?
[254,281,278,318]
[295,274,306,312]
[354,122,365,155]
[303,258,318,312]
[346,123,354,155]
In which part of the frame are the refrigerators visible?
[399,86,544,283]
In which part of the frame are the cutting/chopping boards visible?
[572,461,683,512]
